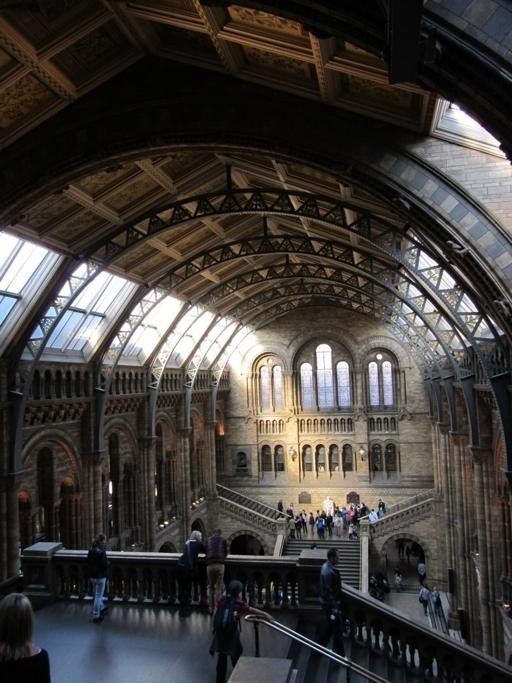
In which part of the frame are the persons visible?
[277,500,284,518]
[314,548,348,661]
[419,584,430,616]
[175,531,206,621]
[86,534,110,624]
[395,574,403,593]
[287,516,300,538]
[323,496,334,515]
[377,506,385,519]
[211,581,274,682]
[295,517,302,538]
[431,587,440,609]
[395,539,416,562]
[0,593,52,683]
[287,502,294,517]
[300,510,308,534]
[378,498,386,514]
[204,528,229,616]
[309,502,369,539]
[369,509,378,521]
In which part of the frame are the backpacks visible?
[213,601,234,633]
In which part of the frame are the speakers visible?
[457,607,468,639]
[447,568,454,594]
